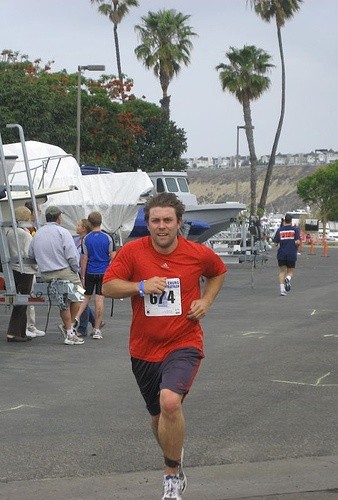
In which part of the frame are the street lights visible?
[235,125,256,199]
[75,64,106,164]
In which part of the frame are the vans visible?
[270,212,310,227]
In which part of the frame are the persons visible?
[273,214,301,296]
[101,192,227,500]
[6,201,117,345]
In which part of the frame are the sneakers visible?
[58,324,67,338]
[91,329,103,339]
[64,334,85,344]
[178,447,188,494]
[162,473,181,500]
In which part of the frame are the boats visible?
[1,140,247,248]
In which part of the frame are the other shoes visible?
[31,329,45,336]
[281,290,287,296]
[25,329,37,338]
[285,278,290,291]
[6,334,32,342]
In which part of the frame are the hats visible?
[45,205,65,215]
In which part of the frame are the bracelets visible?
[139,279,146,296]
[81,277,85,280]
